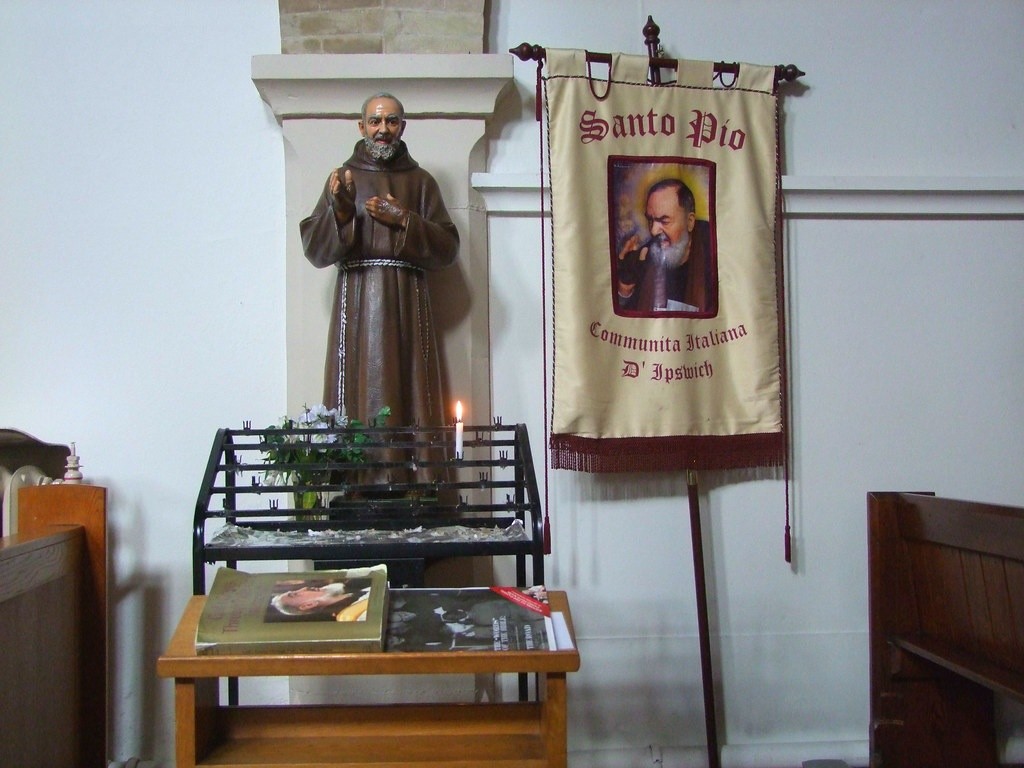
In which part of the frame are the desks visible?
[159,595,581,768]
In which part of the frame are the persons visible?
[271,577,370,622]
[299,91,460,499]
[616,177,711,311]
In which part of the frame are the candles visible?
[456,401,464,459]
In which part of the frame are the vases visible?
[294,479,323,522]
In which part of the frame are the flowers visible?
[261,403,390,485]
